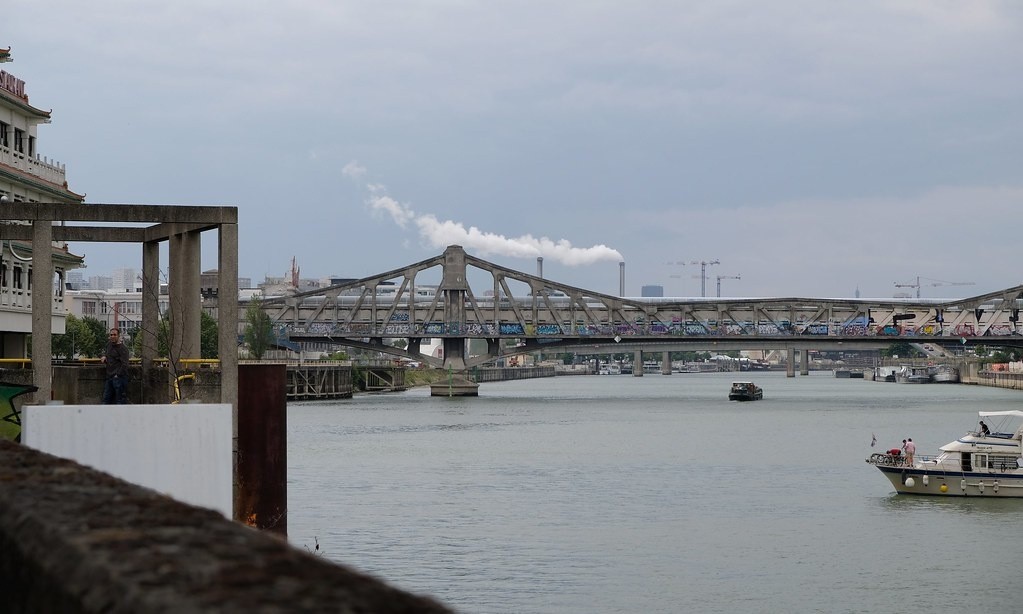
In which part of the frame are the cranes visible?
[662,258,720,297]
[671,273,742,297]
[895,276,975,298]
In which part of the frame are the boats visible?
[750,362,771,370]
[929,364,958,382]
[865,410,1023,497]
[622,368,632,374]
[729,382,763,401]
[895,365,930,384]
[599,364,610,375]
[609,365,621,375]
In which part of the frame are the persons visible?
[886,438,916,467]
[978,421,990,435]
[99,329,140,404]
